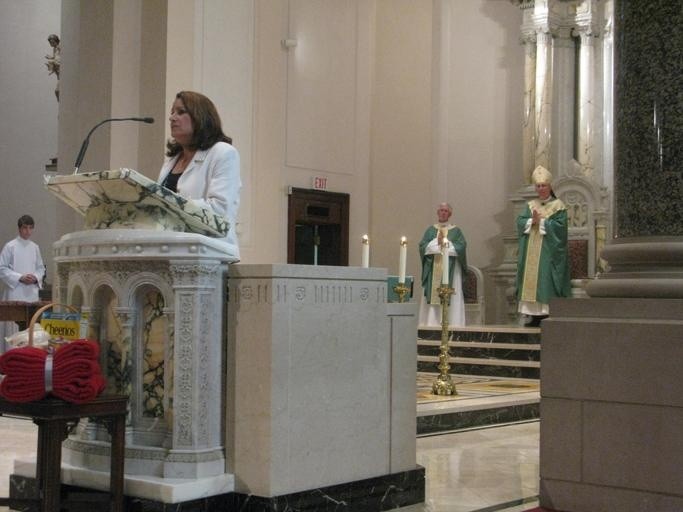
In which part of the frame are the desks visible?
[0,300,53,331]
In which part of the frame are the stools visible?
[0,393,131,512]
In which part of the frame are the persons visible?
[0,214,46,332]
[418,204,467,328]
[517,164,573,325]
[154,90,240,257]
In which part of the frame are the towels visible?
[0,338,106,403]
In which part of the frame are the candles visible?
[360,234,369,266]
[442,236,449,289]
[398,235,407,283]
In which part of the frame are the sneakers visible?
[525,321,541,327]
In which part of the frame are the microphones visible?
[72,117,154,173]
